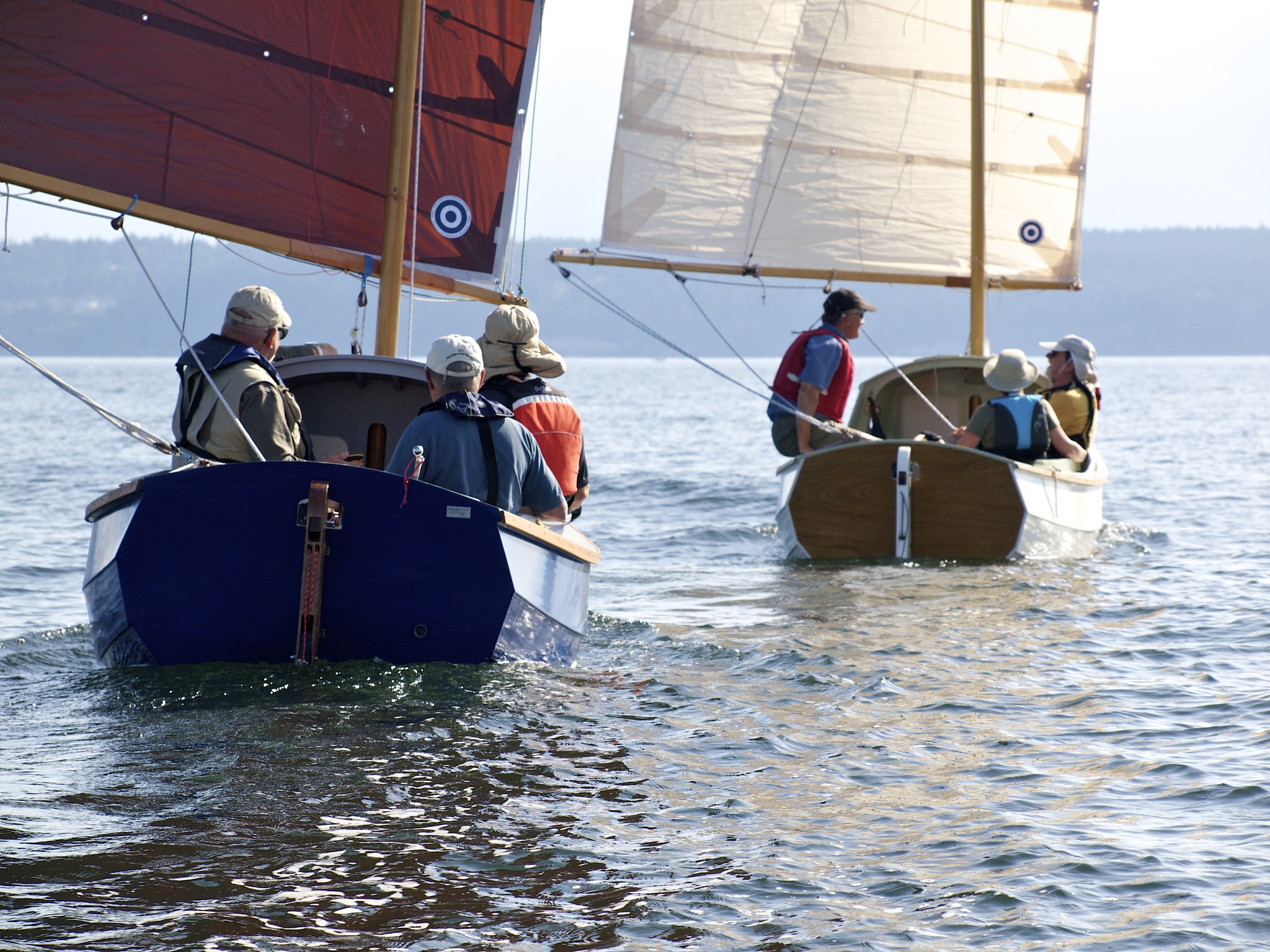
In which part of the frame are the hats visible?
[1039,334,1101,387]
[476,303,565,379]
[983,349,1039,392]
[226,286,292,328]
[823,288,877,315]
[425,334,484,376]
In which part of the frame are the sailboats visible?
[551,0,1113,568]
[0,0,601,670]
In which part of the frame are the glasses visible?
[263,326,289,345]
[1051,350,1069,360]
[842,310,864,320]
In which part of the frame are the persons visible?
[767,289,882,457]
[384,334,568,522]
[947,335,1101,459]
[171,285,365,471]
[476,305,589,511]
[956,349,1086,464]
